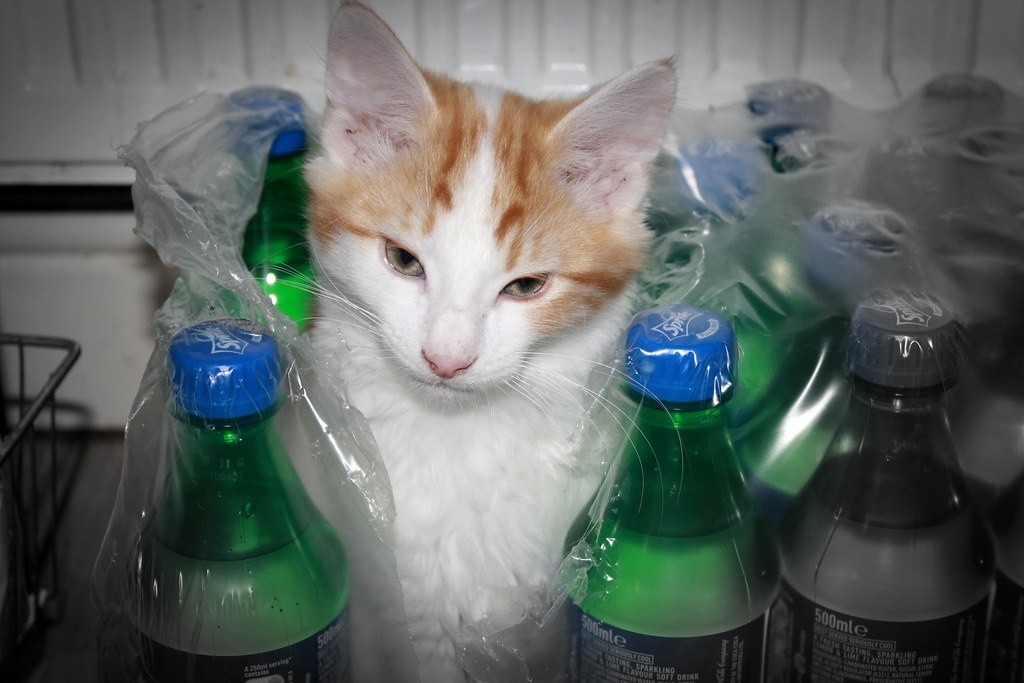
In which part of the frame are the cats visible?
[246,0,681,683]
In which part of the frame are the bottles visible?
[135,64,1024,680]
